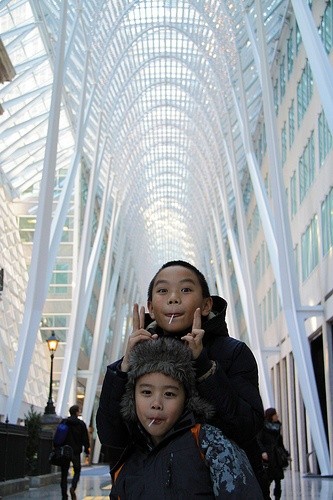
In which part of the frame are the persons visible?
[263,408,289,500]
[96,260,265,480]
[109,338,263,500]
[53,405,90,500]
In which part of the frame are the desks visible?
[0,423,54,483]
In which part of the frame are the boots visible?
[273,488,282,500]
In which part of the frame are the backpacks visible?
[52,419,69,446]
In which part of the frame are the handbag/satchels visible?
[47,445,73,466]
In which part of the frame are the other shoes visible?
[61,496,67,500]
[69,487,76,500]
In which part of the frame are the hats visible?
[117,332,214,422]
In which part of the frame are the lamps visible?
[46,332,60,353]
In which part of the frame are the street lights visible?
[43,330,59,415]
